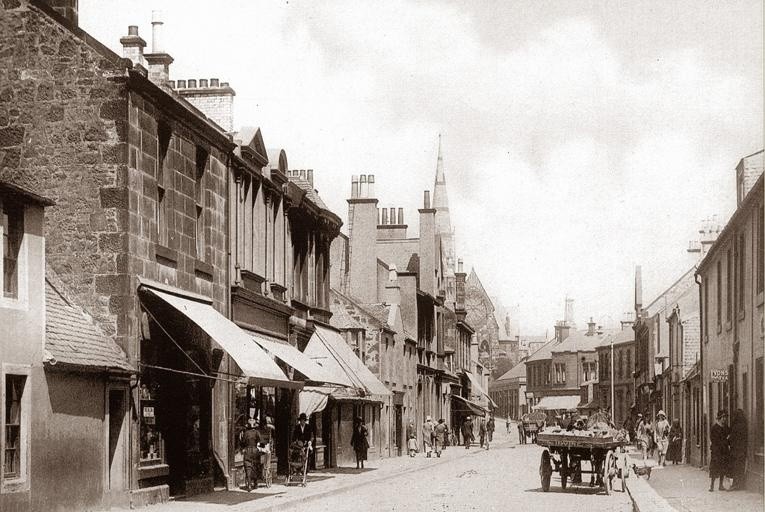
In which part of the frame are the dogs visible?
[631,463,655,480]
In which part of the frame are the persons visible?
[709,410,732,490]
[405,414,498,457]
[727,408,748,492]
[566,407,584,483]
[351,416,370,469]
[560,408,572,429]
[623,409,657,466]
[653,409,671,466]
[289,412,314,480]
[666,417,684,465]
[239,417,263,492]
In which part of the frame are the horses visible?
[566,411,587,483]
[588,405,612,486]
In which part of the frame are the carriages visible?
[517,410,547,445]
[536,403,632,496]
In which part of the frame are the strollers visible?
[286,440,312,487]
[245,441,275,488]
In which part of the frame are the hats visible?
[297,412,309,421]
[354,416,365,423]
[426,415,446,424]
[716,408,745,419]
[408,434,416,439]
[656,410,666,419]
